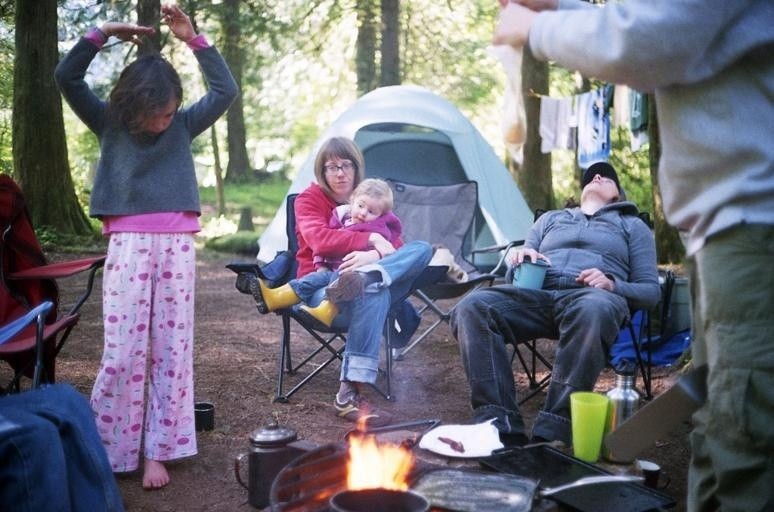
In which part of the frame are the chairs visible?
[273,192,401,406]
[377,177,527,364]
[504,208,658,406]
[0,165,111,399]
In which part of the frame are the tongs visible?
[342,416,442,451]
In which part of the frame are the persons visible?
[1,385,126,512]
[249,178,397,329]
[52,1,237,491]
[481,0,773,510]
[291,136,436,424]
[455,161,663,448]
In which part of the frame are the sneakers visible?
[325,272,363,304]
[334,392,393,428]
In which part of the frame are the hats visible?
[582,162,626,201]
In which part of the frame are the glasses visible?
[323,164,356,176]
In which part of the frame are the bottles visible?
[605,359,639,466]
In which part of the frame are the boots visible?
[298,299,340,329]
[250,278,300,314]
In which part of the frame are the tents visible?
[256,83,536,280]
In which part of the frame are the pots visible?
[407,468,650,512]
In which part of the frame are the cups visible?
[511,259,548,290]
[569,392,609,464]
[233,420,299,508]
[330,488,428,512]
[631,460,670,493]
[195,402,215,432]
